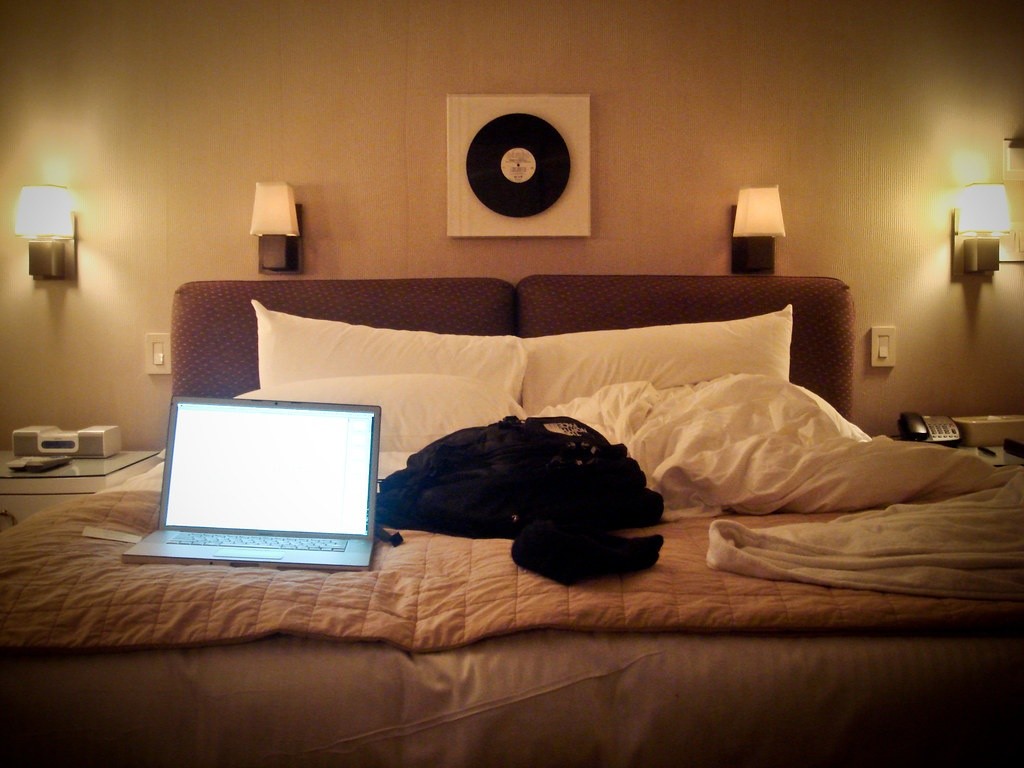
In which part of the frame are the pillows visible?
[519,303,794,419]
[229,375,528,451]
[248,299,529,404]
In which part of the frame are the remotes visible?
[7,456,75,474]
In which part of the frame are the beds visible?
[0,280,1024,768]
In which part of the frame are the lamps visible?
[954,182,1012,278]
[726,186,787,276]
[15,183,79,281]
[247,180,308,276]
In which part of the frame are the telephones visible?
[897,411,960,443]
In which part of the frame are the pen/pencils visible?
[978,447,995,456]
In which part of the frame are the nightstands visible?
[1,450,161,536]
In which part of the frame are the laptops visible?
[121,397,383,575]
